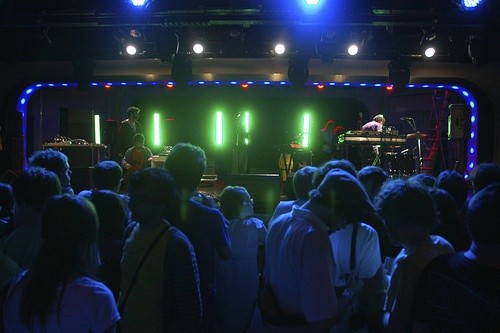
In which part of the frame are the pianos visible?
[344,130,413,146]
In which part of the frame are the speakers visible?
[447,104,470,141]
[0,112,22,153]
[228,173,281,214]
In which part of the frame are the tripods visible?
[453,140,471,180]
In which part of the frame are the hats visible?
[319,167,376,212]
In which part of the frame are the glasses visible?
[243,199,254,205]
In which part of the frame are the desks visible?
[40,143,104,188]
[342,136,407,179]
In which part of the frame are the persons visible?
[362,113,385,164]
[116,106,144,158]
[0,126,500,333]
